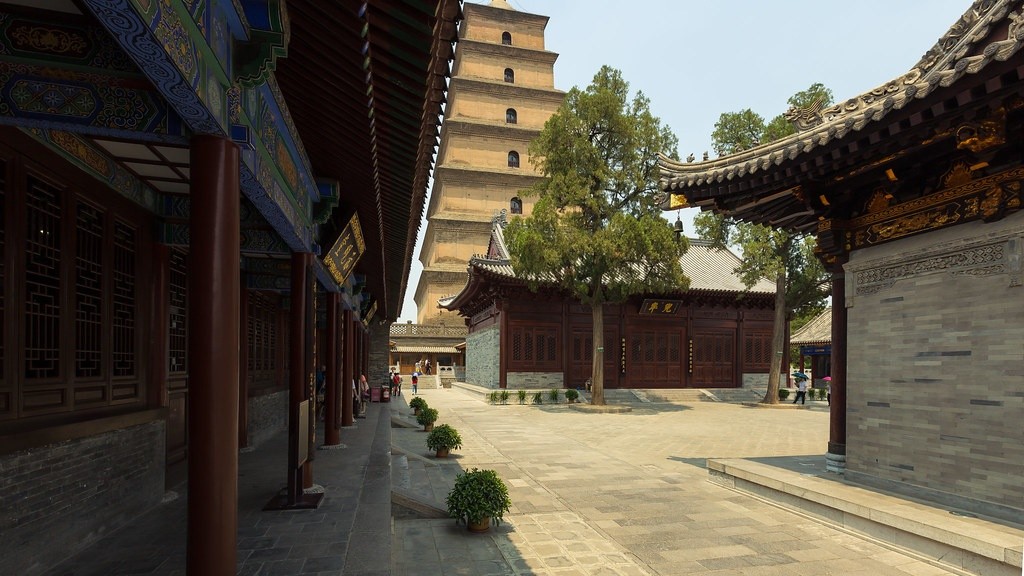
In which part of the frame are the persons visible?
[793,378,808,404]
[316,365,326,420]
[359,375,369,413]
[415,357,432,375]
[412,373,418,395]
[827,381,831,406]
[352,379,357,423]
[389,367,403,396]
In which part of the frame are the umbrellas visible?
[791,373,809,379]
[822,377,831,381]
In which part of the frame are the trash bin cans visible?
[381,385,390,403]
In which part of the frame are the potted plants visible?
[409,397,428,416]
[779,390,790,401]
[416,408,439,431]
[425,424,463,457]
[565,389,578,404]
[446,468,512,533]
